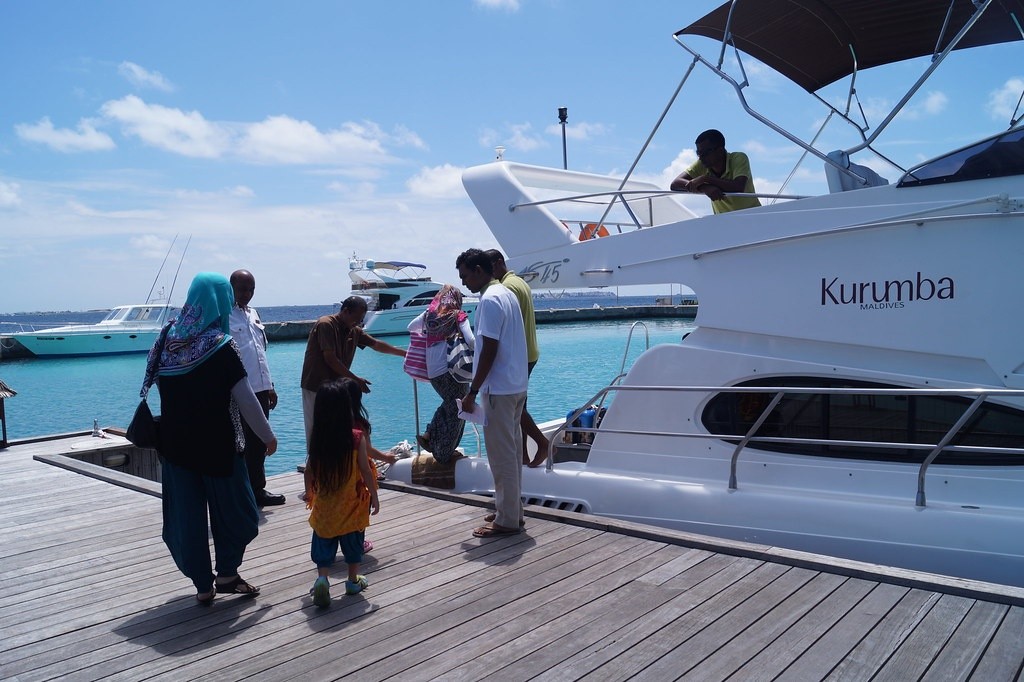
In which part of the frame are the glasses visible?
[695,148,719,157]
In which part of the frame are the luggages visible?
[564,403,608,444]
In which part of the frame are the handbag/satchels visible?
[125,321,176,451]
[447,309,475,384]
[402,307,431,382]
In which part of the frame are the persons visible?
[300,295,409,499]
[453,250,527,539]
[669,130,762,215]
[486,249,557,468]
[335,376,401,554]
[302,382,381,605]
[145,272,277,604]
[226,268,287,505]
[403,285,477,466]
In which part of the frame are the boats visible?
[381,0,1024,590]
[0,234,195,358]
[333,250,490,334]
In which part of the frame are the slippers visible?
[214,575,261,594]
[195,588,217,606]
[472,524,523,537]
[483,512,526,527]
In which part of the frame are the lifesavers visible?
[579,224,611,241]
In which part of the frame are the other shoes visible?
[363,539,373,553]
[447,449,464,462]
[345,574,369,595]
[415,435,435,452]
[312,575,331,608]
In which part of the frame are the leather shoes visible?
[255,490,286,506]
